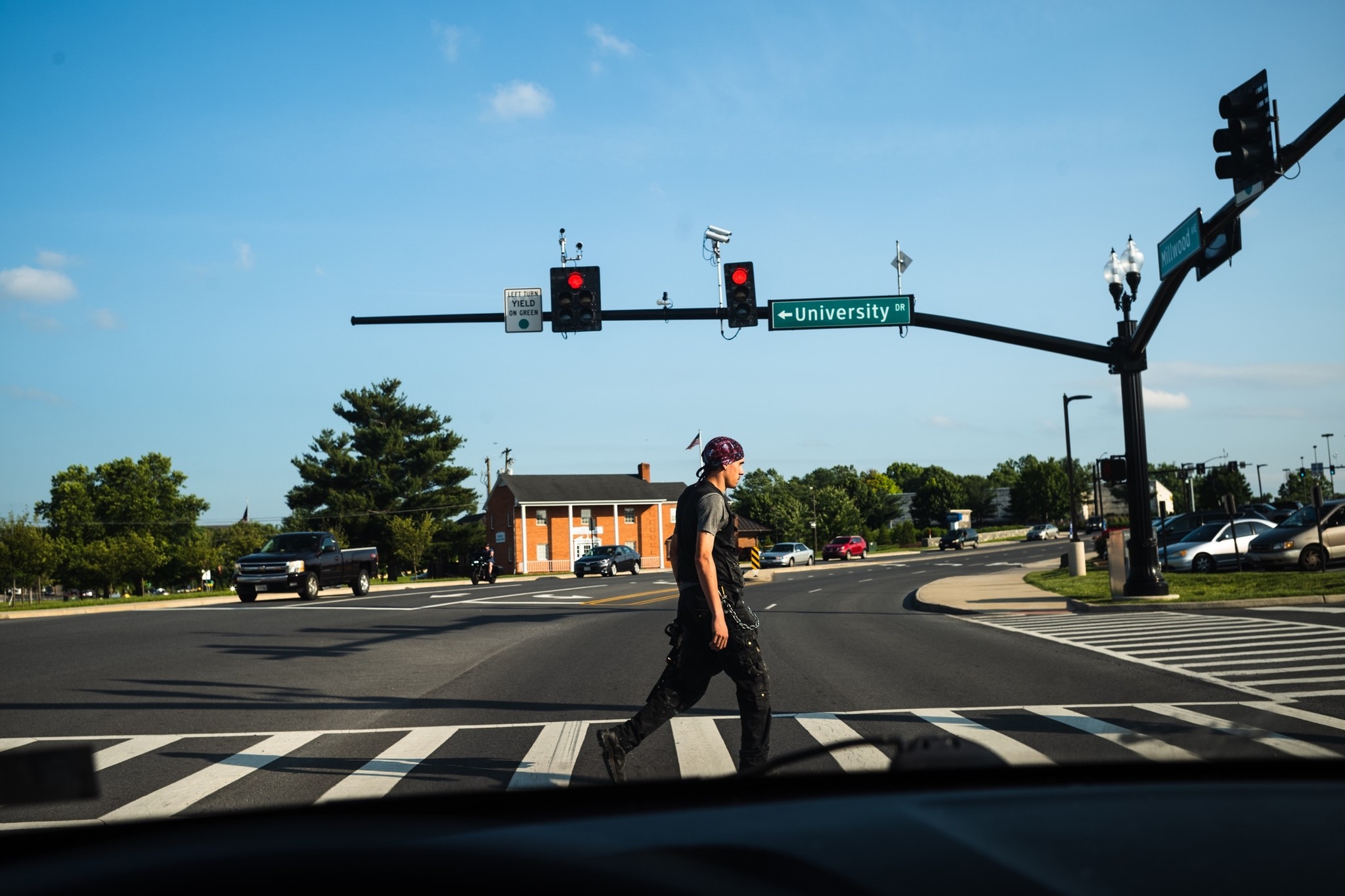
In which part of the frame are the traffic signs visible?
[770,294,913,330]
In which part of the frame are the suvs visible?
[821,535,868,561]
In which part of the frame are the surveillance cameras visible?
[705,226,733,243]
[559,228,583,256]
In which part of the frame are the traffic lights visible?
[1300,467,1305,477]
[550,265,603,333]
[723,261,758,328]
[1330,465,1335,475]
[1212,67,1277,194]
[1100,458,1126,482]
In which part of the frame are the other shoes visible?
[596,727,626,784]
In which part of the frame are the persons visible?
[479,543,495,577]
[597,437,773,782]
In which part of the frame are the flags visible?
[685,432,701,450]
[241,506,249,521]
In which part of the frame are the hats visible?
[701,436,744,467]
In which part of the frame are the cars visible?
[1125,497,1345,574]
[573,545,641,578]
[1026,523,1059,541]
[939,527,978,551]
[759,541,815,570]
[1084,516,1109,534]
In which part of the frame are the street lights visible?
[1321,433,1334,499]
[1257,464,1267,500]
[1181,462,1194,512]
[1283,468,1291,500]
[1061,391,1092,541]
[809,486,818,558]
[1101,232,1170,597]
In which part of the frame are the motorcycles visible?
[470,554,504,585]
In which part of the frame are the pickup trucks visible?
[234,530,381,603]
